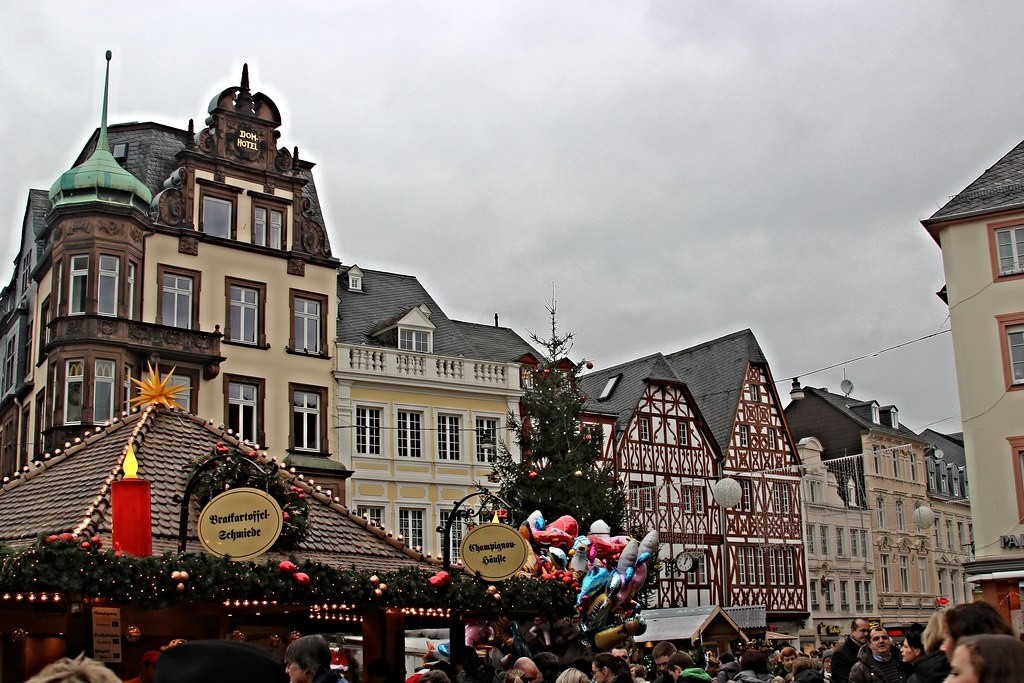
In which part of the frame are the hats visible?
[157,640,285,683]
[142,650,160,664]
[822,649,834,668]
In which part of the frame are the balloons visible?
[412,508,659,672]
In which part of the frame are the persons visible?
[24,597,1024,683]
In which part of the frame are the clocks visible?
[675,550,699,573]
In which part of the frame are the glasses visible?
[591,669,600,675]
[617,656,628,659]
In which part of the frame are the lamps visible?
[789,378,805,400]
[645,641,655,648]
[481,428,493,449]
